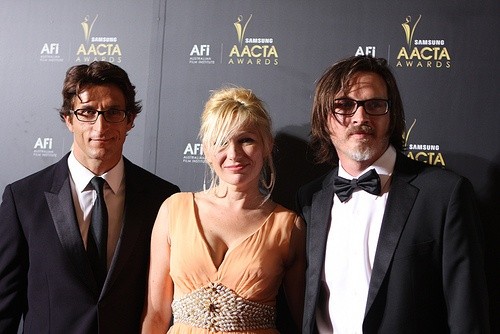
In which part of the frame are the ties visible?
[87,176,110,294]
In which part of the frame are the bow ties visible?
[332,169,381,203]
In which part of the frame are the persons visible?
[141,87,307,334]
[0,61,182,334]
[293,55,490,334]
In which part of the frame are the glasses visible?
[330,98,392,116]
[70,109,130,123]
